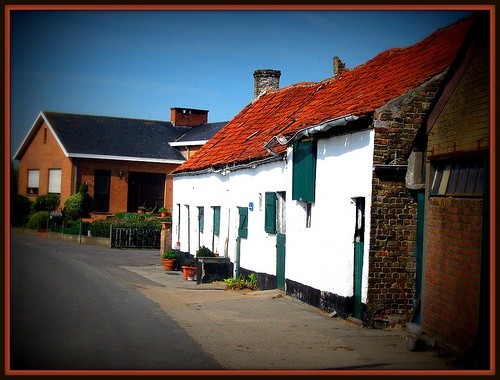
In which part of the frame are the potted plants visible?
[181,259,197,280]
[137,207,145,214]
[161,250,180,271]
[160,216,172,229]
[158,207,168,217]
